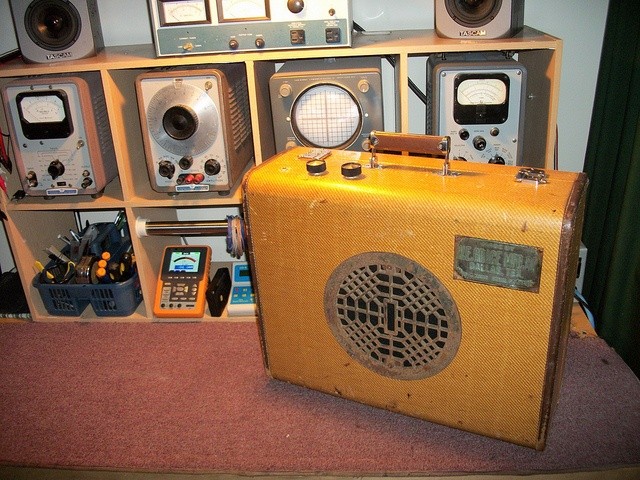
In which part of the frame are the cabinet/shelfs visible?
[0,25,563,321]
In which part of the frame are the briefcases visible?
[242,130,590,450]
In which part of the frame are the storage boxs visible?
[32,223,139,316]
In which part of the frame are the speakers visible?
[9,0,106,63]
[434,0,525,40]
